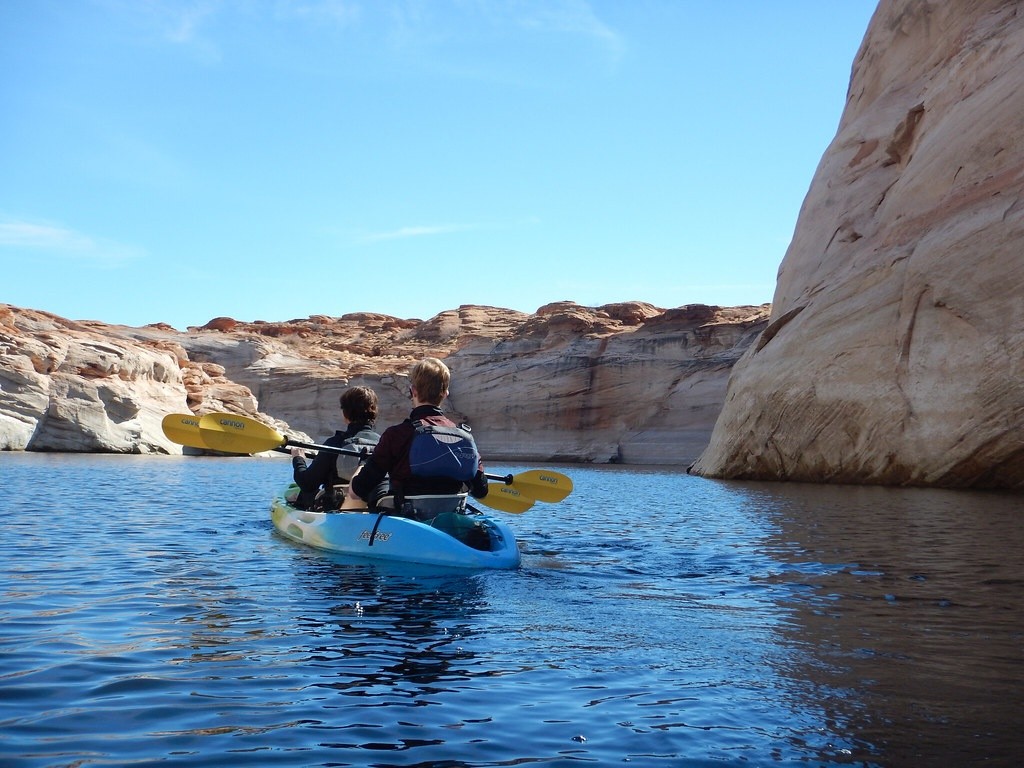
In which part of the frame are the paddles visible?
[162,412,535,513]
[197,412,572,502]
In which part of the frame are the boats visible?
[270,482,525,571]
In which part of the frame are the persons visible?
[350,357,488,521]
[291,386,390,513]
